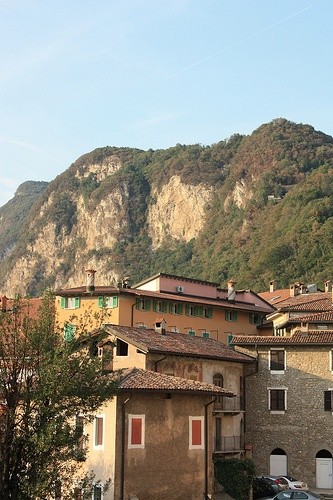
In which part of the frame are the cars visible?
[261,488,325,500]
[277,475,309,490]
[253,480,286,498]
[253,476,286,490]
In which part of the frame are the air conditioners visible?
[176,286,185,292]
